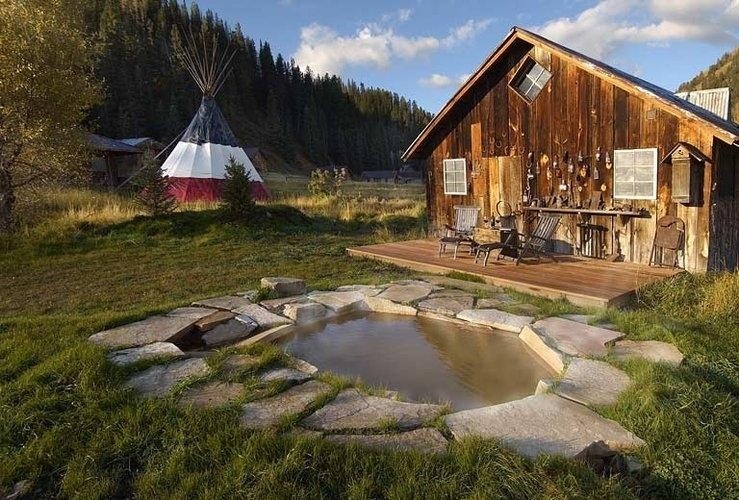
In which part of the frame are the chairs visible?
[437,205,483,260]
[647,215,687,271]
[471,216,562,267]
